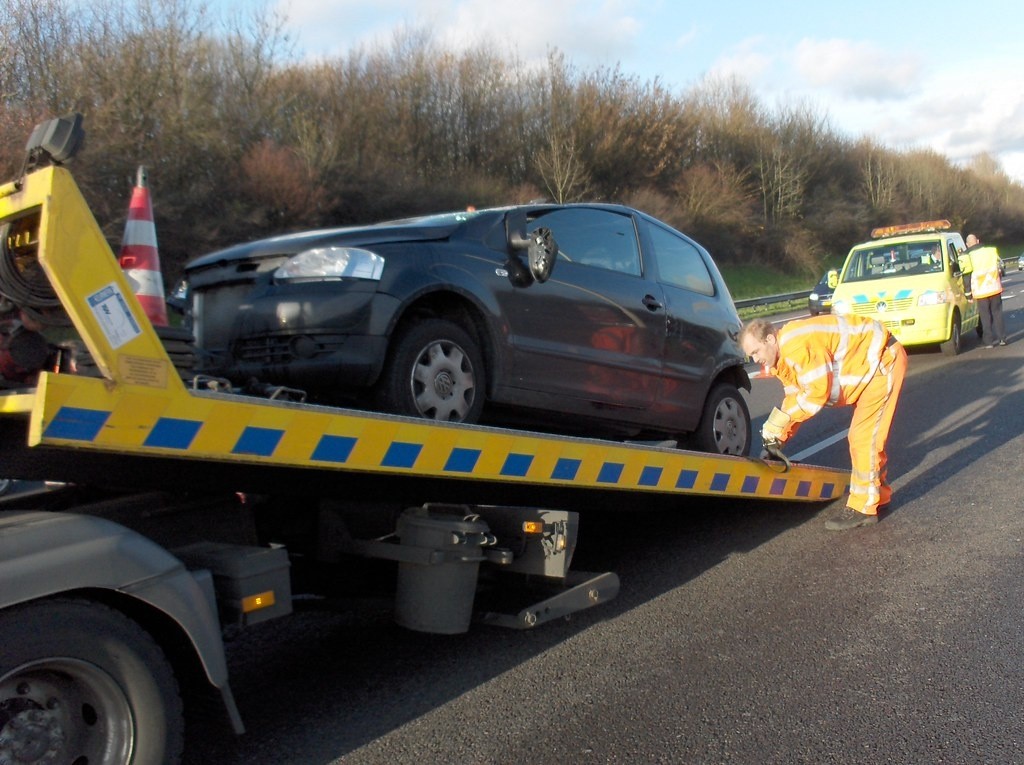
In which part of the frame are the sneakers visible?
[825,507,878,530]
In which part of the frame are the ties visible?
[936,259,939,266]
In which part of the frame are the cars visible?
[1018,252,1024,270]
[997,256,1007,278]
[167,202,754,458]
[807,269,868,317]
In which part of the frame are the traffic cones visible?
[118,165,168,329]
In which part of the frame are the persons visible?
[740,315,908,532]
[958,234,1007,349]
[0,295,70,392]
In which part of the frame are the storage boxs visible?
[168,541,293,624]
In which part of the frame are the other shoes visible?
[999,340,1006,345]
[983,344,994,349]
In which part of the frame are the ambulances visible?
[828,220,983,356]
